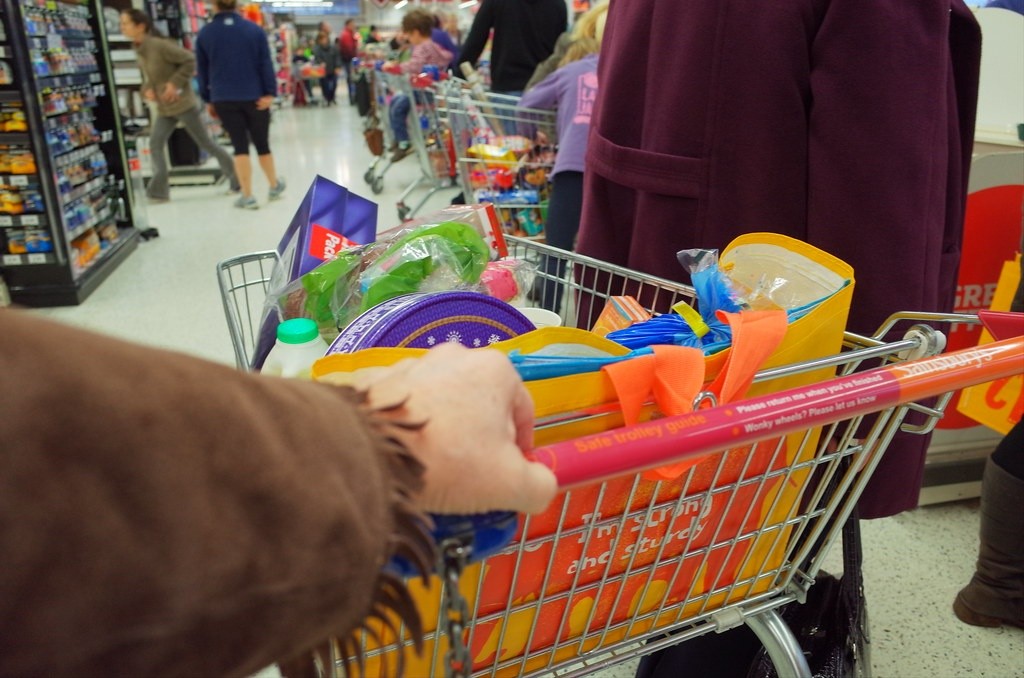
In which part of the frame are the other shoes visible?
[269,175,287,200]
[226,184,242,195]
[233,196,258,209]
[145,193,170,201]
[452,192,466,204]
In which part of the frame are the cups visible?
[515,307,561,327]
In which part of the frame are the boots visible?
[954,455,1024,631]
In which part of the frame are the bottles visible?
[258,317,330,383]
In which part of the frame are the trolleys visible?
[397,74,522,222]
[440,72,562,248]
[353,58,391,121]
[364,61,440,192]
[292,62,324,79]
[216,232,1024,678]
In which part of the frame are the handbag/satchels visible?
[365,119,384,156]
[635,567,873,678]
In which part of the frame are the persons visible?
[120,0,612,311]
[576,0,982,678]
[0,307,559,678]
[954,411,1023,629]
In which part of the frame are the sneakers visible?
[389,140,414,162]
